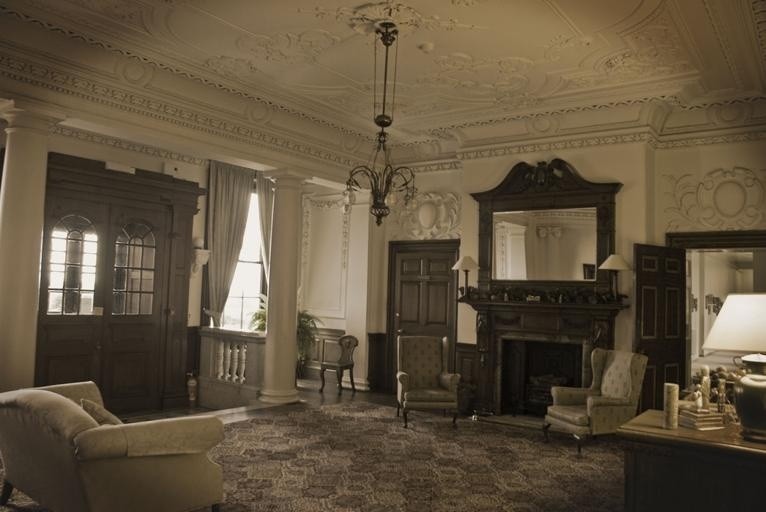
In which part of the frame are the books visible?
[678,409,724,431]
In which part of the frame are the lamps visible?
[342,22,416,228]
[597,253,632,303]
[191,234,211,274]
[450,256,482,300]
[702,292,766,442]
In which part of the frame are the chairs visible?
[395,335,461,430]
[318,335,359,396]
[541,348,651,455]
[0,380,226,512]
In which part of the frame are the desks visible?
[617,408,766,512]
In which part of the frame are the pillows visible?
[80,398,124,426]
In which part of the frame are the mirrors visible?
[467,157,624,303]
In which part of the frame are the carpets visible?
[213,399,626,512]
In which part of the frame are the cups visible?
[663,382,679,430]
